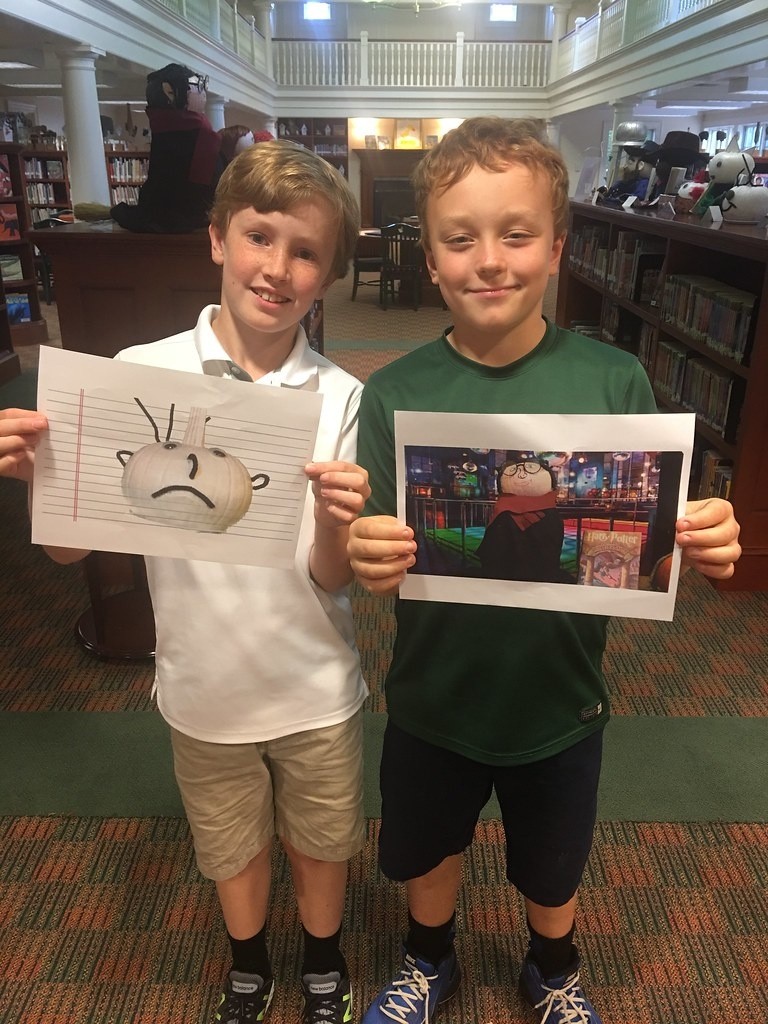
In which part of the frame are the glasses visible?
[188,74,210,93]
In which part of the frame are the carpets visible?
[0,718,766,819]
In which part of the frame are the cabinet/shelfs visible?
[303,1,331,20]
[28,150,150,219]
[490,4,517,21]
[278,117,349,182]
[555,197,768,591]
[0,141,48,346]
[352,147,439,230]
[0,268,22,386]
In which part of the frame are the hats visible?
[624,139,658,163]
[653,131,708,166]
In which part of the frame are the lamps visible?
[360,0,476,17]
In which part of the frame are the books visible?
[24,135,150,228]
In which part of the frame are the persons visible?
[347,118,741,1024]
[647,468,681,593]
[0,139,372,1024]
[607,131,702,200]
[755,177,763,186]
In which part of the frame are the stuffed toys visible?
[110,63,276,233]
[475,458,565,580]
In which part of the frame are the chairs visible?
[352,223,424,311]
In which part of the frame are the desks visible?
[359,224,449,312]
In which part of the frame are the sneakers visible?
[518,950,601,1024]
[361,943,462,1024]
[300,949,353,1024]
[213,961,274,1024]
